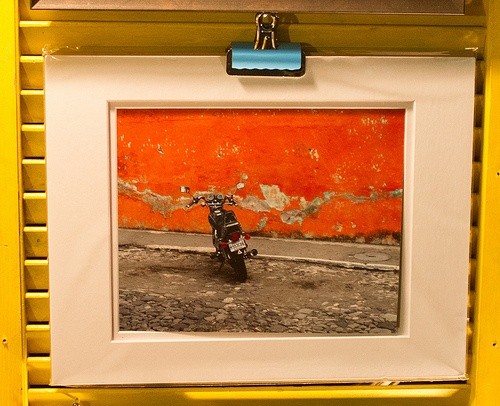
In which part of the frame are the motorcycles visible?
[179,181,258,282]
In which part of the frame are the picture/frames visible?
[42,49,479,389]
